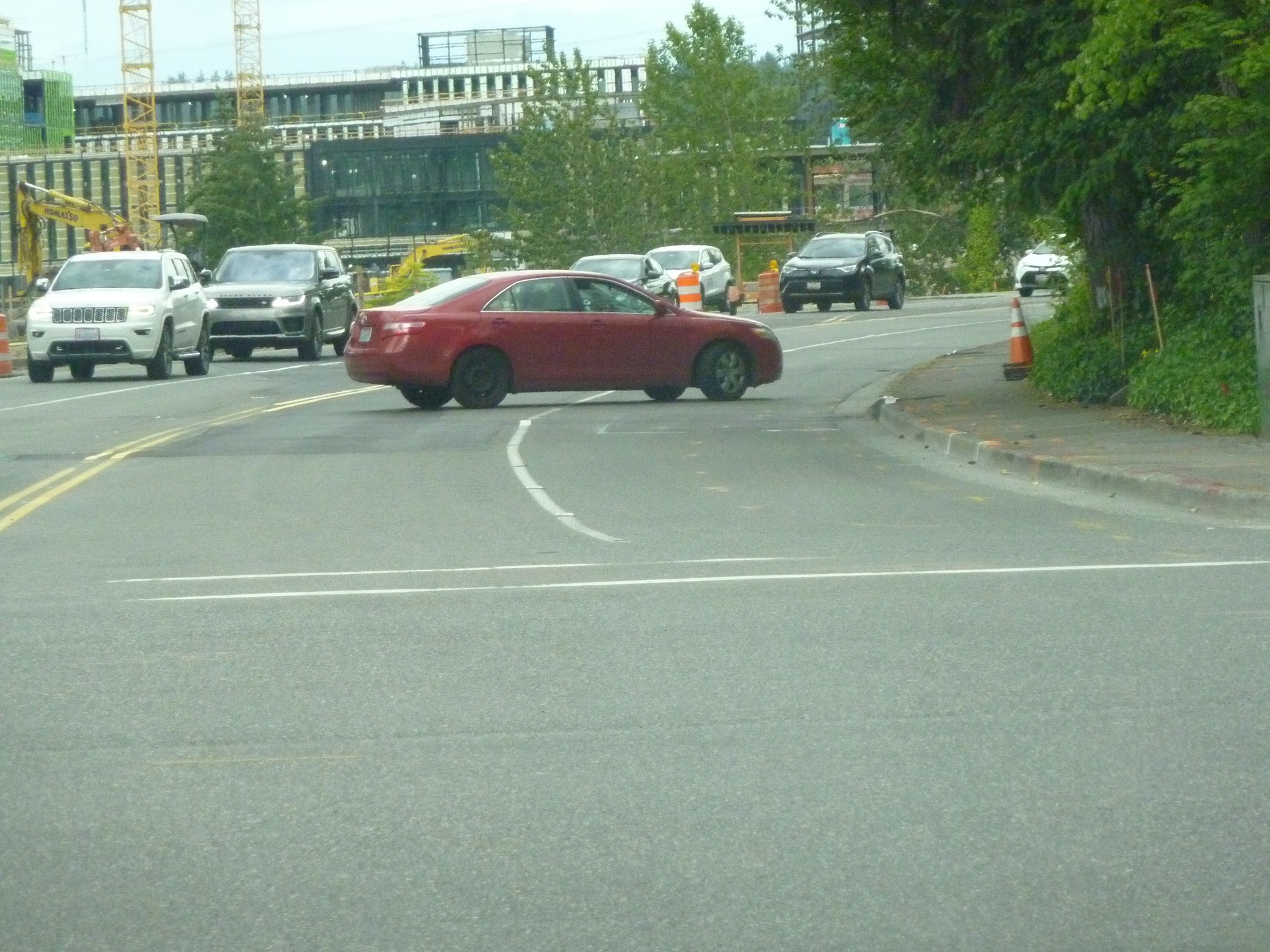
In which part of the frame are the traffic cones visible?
[1002,298,1034,381]
[0,313,25,378]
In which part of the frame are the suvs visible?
[645,245,734,313]
[26,249,213,382]
[203,244,358,362]
[778,230,906,313]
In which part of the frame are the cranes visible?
[230,0,264,125]
[119,0,162,249]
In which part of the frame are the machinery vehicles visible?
[354,229,494,310]
[16,180,209,311]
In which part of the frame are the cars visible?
[342,269,785,408]
[1014,233,1078,297]
[569,254,680,313]
